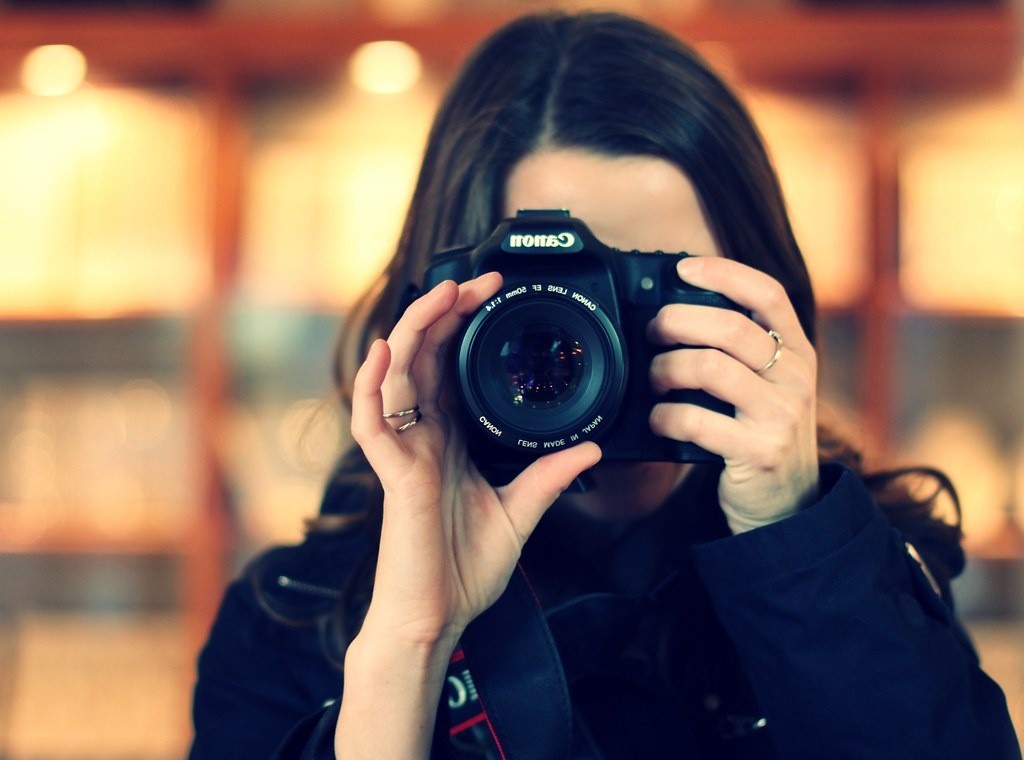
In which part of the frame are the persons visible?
[189,10,1022,760]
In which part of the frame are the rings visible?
[383,406,423,433]
[754,331,782,374]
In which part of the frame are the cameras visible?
[410,210,736,469]
